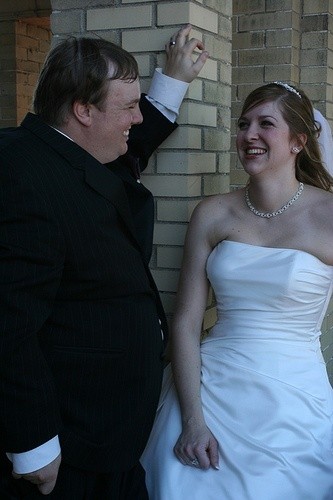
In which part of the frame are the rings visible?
[190,458,198,466]
[170,42,176,46]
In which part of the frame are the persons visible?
[139,82,333,500]
[1,23,208,500]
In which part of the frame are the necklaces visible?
[245,181,304,218]
